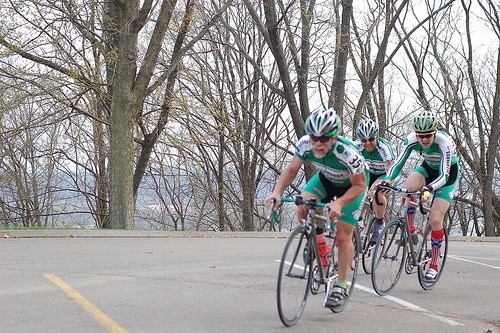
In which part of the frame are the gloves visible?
[420,184,434,197]
[374,182,391,194]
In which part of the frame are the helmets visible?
[304,108,343,138]
[411,111,438,135]
[356,118,379,139]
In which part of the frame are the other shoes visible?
[322,246,333,276]
[370,223,386,243]
[422,267,440,284]
[302,242,317,265]
[323,285,350,309]
[395,232,419,246]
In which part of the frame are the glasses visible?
[309,135,330,143]
[417,134,434,139]
[360,137,376,142]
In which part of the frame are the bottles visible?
[409,225,419,246]
[322,239,334,265]
[316,225,326,254]
[414,230,423,253]
[358,217,364,232]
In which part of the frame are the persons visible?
[353,119,402,245]
[264,107,365,308]
[381,110,460,279]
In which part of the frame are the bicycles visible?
[372,183,449,296]
[261,191,389,327]
